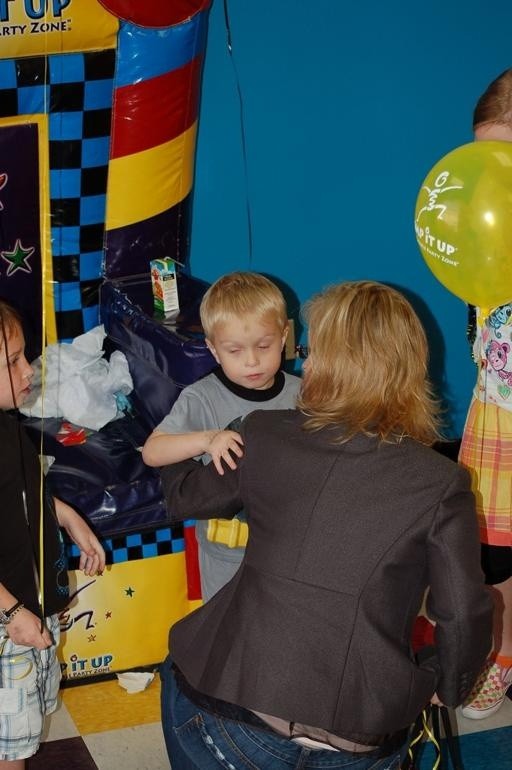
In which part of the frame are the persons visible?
[0,303,106,770]
[458,70,512,720]
[159,279,493,769]
[143,273,303,607]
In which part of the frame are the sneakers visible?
[461,659,512,719]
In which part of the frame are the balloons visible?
[416,140,512,307]
